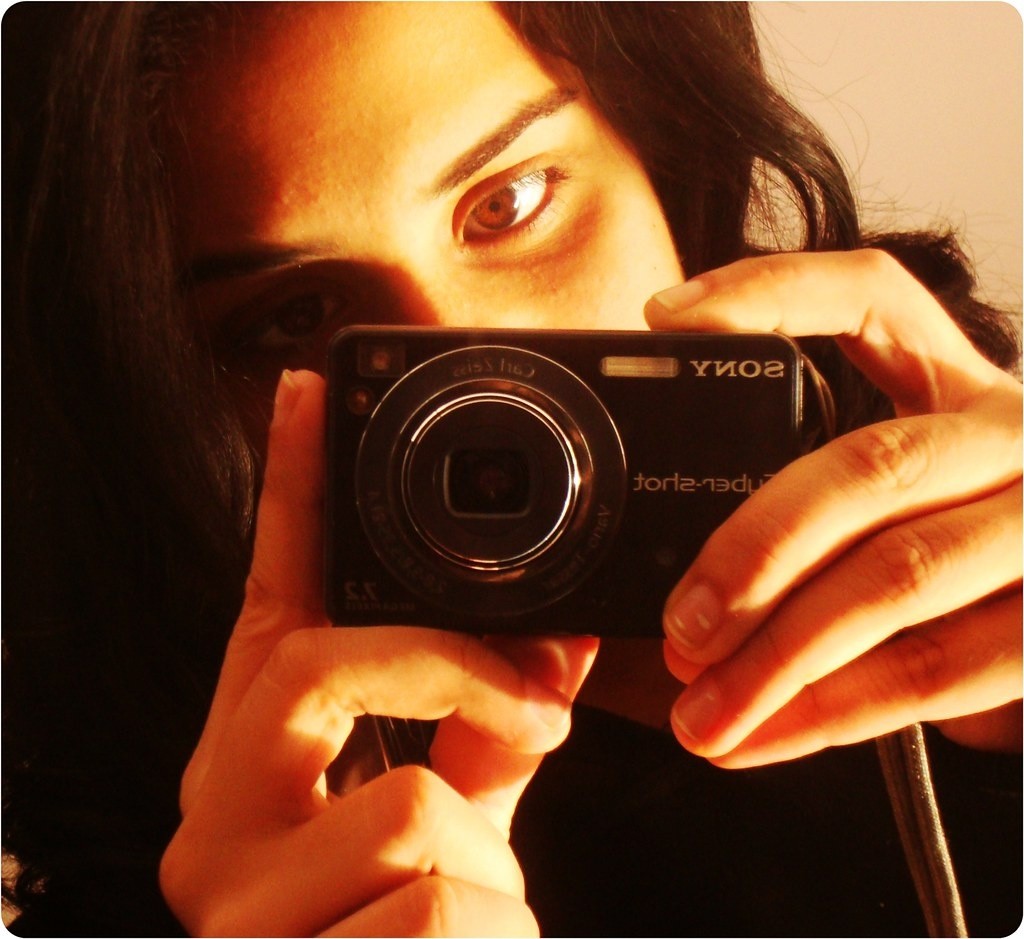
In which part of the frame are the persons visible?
[1,1,1022,939]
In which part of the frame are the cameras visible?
[309,323,807,639]
